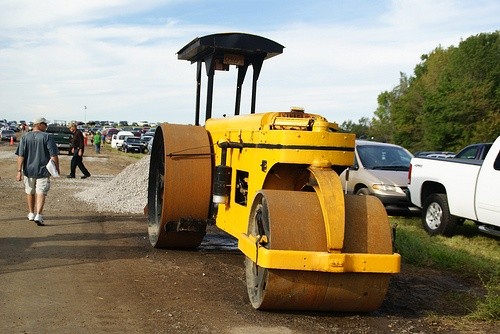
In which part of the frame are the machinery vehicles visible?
[145,32,402,313]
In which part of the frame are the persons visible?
[82,129,107,154]
[14,117,60,224]
[67,123,90,178]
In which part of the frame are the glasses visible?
[40,122,47,127]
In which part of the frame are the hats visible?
[33,117,50,123]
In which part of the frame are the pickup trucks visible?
[45,125,74,155]
[408,133,500,238]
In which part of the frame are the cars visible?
[77,124,156,154]
[0,119,34,134]
[1,130,17,142]
[413,150,475,159]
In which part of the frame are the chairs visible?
[386,151,401,163]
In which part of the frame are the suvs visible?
[339,140,422,211]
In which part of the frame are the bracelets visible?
[17,170,21,172]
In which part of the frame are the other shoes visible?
[34,215,44,226]
[27,213,34,220]
[66,175,76,178]
[81,174,91,179]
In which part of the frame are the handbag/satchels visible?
[46,159,60,178]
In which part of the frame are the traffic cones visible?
[9,136,14,146]
